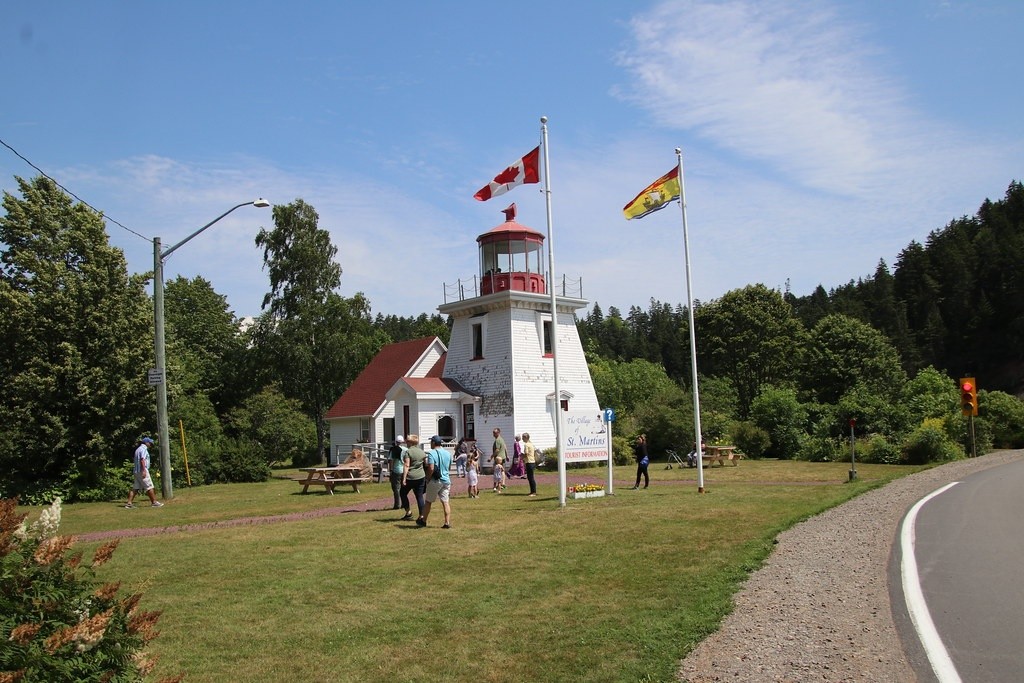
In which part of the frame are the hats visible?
[142,437,153,443]
[429,436,442,445]
[396,435,404,443]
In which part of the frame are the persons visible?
[631,435,649,490]
[507,435,526,480]
[493,457,504,493]
[125,437,164,509]
[454,437,467,478]
[470,443,479,470]
[416,435,452,529]
[466,453,479,498]
[519,433,537,497]
[400,434,427,520]
[387,435,408,510]
[487,427,509,489]
[594,415,606,434]
[688,435,706,468]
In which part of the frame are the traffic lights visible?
[960,377,978,417]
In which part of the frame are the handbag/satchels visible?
[432,450,442,480]
[640,456,649,470]
[514,457,520,464]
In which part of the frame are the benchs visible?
[702,454,745,458]
[291,477,371,485]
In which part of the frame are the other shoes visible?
[506,473,511,479]
[393,507,399,509]
[474,495,479,498]
[458,476,460,478]
[644,486,649,490]
[498,491,501,494]
[470,495,475,498]
[521,477,526,479]
[125,504,136,509]
[416,519,426,527]
[493,488,496,492]
[152,501,164,507]
[441,524,451,529]
[528,493,536,497]
[633,486,638,490]
[462,476,465,478]
[401,513,413,520]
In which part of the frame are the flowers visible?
[569,484,604,492]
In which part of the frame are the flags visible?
[473,142,542,202]
[622,164,682,223]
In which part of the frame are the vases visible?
[586,491,598,498]
[568,492,585,499]
[596,490,605,497]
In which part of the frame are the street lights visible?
[151,197,270,499]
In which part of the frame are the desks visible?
[299,466,362,495]
[707,446,738,467]
